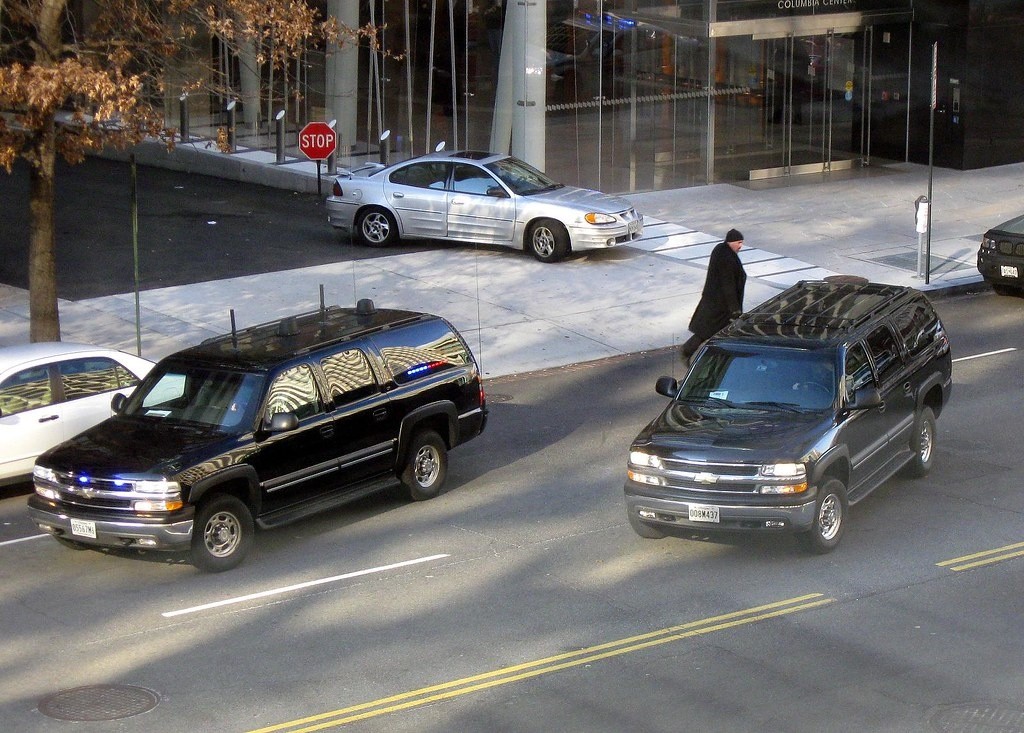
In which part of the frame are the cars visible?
[1,340,186,489]
[976,213,1024,299]
[325,149,644,263]
[548,8,704,106]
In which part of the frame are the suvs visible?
[26,297,491,574]
[625,274,953,554]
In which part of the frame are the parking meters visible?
[913,194,928,276]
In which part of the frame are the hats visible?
[726,229,744,242]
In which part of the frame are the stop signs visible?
[298,121,337,162]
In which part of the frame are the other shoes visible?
[678,348,690,369]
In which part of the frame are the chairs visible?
[405,166,429,188]
[184,385,222,423]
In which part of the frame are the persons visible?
[793,359,854,392]
[678,228,747,368]
[767,37,810,125]
